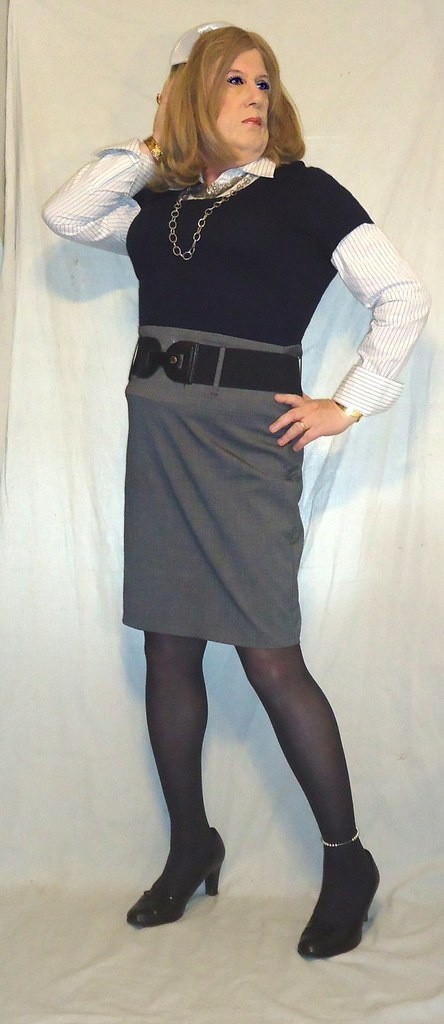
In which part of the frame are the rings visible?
[300,421,306,431]
[156,92,161,104]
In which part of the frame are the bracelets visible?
[336,402,362,423]
[143,136,165,166]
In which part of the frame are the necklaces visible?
[168,174,258,261]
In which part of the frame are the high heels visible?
[298,849,380,959]
[126,827,225,927]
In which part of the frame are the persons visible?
[43,26,432,958]
